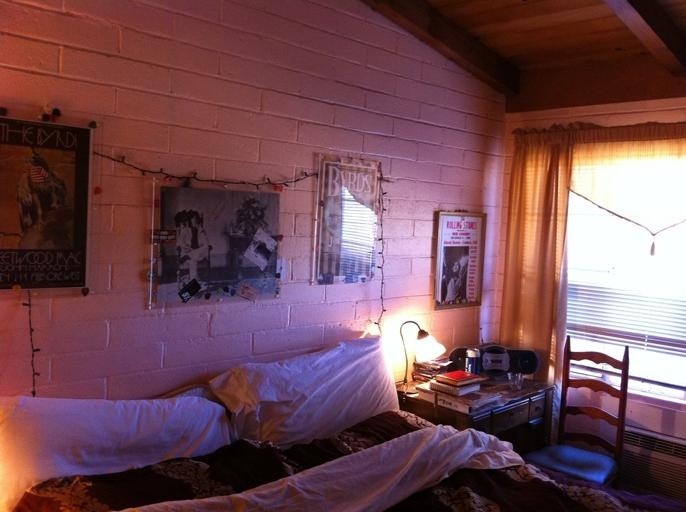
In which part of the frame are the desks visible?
[397,376,557,450]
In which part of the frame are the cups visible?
[507,371,524,392]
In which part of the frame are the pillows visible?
[1,336,399,491]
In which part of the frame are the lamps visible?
[397,318,447,395]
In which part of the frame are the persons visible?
[28,154,50,221]
[175,210,210,283]
[441,256,467,302]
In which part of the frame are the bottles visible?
[465,347,481,376]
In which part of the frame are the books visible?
[410,354,504,415]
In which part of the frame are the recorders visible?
[449,344,539,378]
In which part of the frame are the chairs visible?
[521,334,631,490]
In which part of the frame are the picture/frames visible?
[433,209,487,314]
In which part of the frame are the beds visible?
[0,383,685,509]
